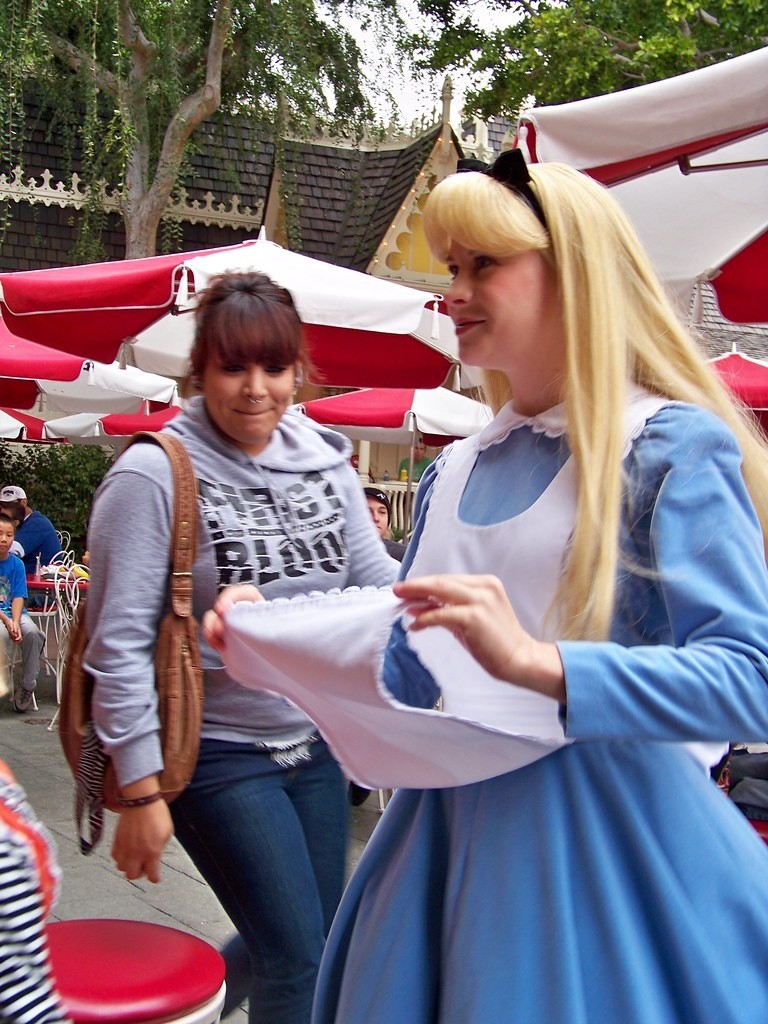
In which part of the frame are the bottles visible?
[400,470,407,482]
[383,470,389,481]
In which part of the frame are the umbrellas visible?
[0,232,496,455]
[521,42,768,321]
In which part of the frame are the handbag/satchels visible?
[57,426,205,813]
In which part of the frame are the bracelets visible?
[117,790,165,810]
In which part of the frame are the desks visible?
[19,572,89,592]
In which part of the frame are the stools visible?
[39,918,226,1024]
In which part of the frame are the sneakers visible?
[13,685,32,713]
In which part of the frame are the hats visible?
[363,486,391,525]
[0,486,27,502]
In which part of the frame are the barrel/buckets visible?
[42,918,227,1024]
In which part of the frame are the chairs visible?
[8,529,92,732]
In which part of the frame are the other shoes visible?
[348,782,371,807]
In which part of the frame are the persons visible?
[86,272,409,1024]
[1,486,63,714]
[203,160,768,1024]
[1,752,71,1023]
